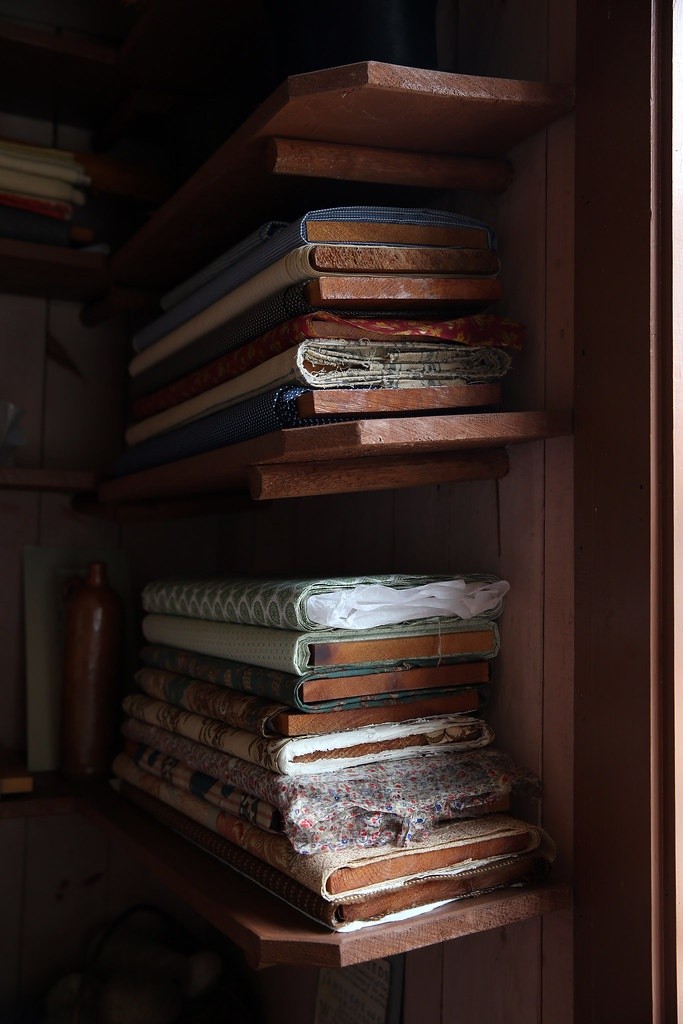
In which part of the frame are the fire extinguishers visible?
[61,559,128,785]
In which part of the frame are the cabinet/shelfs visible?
[84,61,575,972]
[0,237,98,823]
